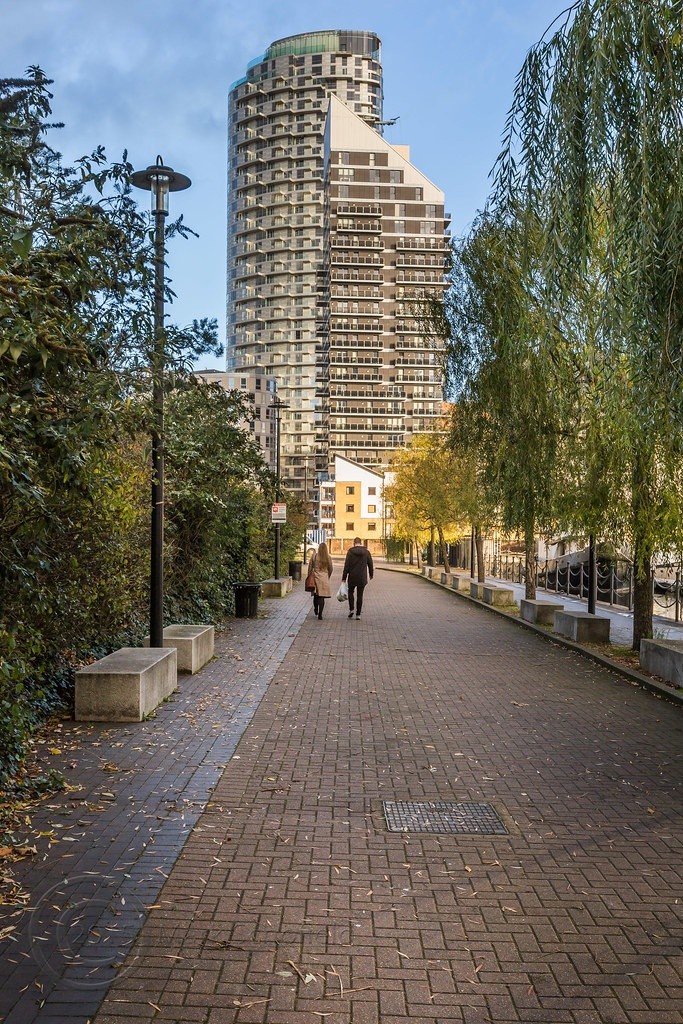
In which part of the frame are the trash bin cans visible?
[289,560,302,581]
[232,583,262,619]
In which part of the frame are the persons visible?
[308,542,333,620]
[342,536,374,620]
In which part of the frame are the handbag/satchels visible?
[337,580,348,601]
[305,573,315,591]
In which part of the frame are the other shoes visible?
[314,609,318,616]
[356,615,361,620]
[318,616,322,620]
[348,610,354,617]
